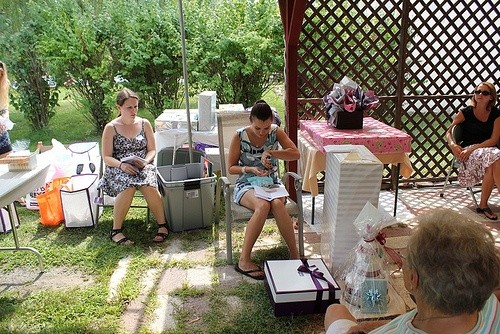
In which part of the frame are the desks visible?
[297,133,414,225]
[0,158,50,273]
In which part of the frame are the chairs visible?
[214,111,303,265]
[440,125,496,209]
[95,161,150,231]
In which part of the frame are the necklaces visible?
[413,316,455,320]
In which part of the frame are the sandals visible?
[153,224,169,241]
[109,229,134,245]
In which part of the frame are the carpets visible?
[302,189,500,320]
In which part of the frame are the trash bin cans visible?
[155,162,218,233]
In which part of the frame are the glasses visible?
[474,90,489,95]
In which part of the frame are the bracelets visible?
[241,166,248,174]
[119,162,122,169]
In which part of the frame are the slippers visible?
[481,207,497,220]
[235,265,265,280]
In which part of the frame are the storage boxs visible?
[60,174,104,229]
[0,202,21,233]
[154,92,247,234]
[321,144,384,276]
[326,108,363,128]
[299,119,411,153]
[0,152,37,170]
[264,259,342,318]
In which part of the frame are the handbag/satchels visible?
[37,177,74,226]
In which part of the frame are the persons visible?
[228,100,300,279]
[102,88,169,245]
[325,207,500,334]
[0,61,27,207]
[446,82,500,220]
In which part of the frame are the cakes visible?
[344,245,390,307]
